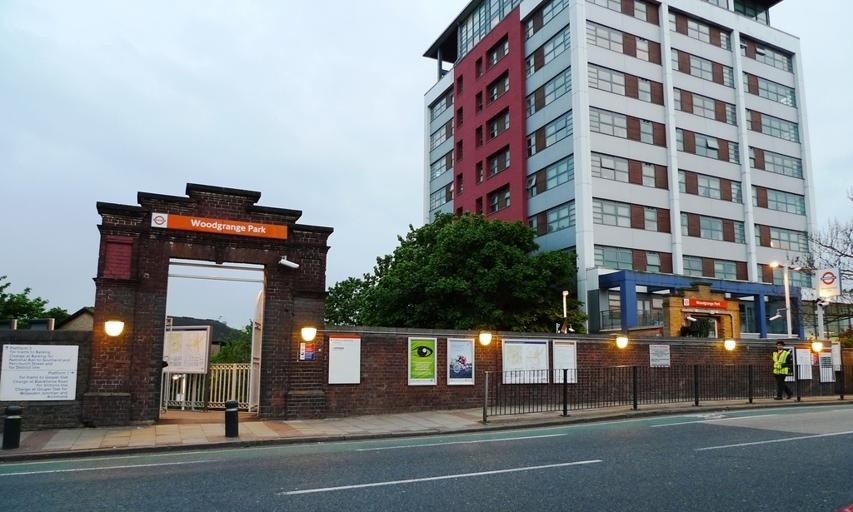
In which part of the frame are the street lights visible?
[770,261,801,339]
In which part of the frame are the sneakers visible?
[774,392,794,400]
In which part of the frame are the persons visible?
[771,341,793,400]
